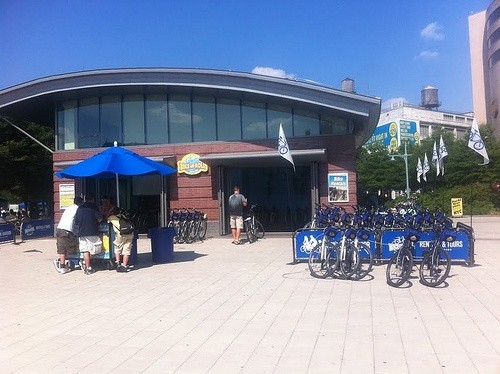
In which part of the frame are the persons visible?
[228,186,247,245]
[97,196,117,218]
[107,207,134,271]
[73,194,104,275]
[53,197,83,274]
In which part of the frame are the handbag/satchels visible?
[441,229,457,242]
[357,229,370,240]
[405,229,420,242]
[324,227,339,238]
[315,209,445,224]
[345,228,356,239]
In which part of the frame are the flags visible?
[468,116,490,165]
[416,136,448,183]
[278,123,296,173]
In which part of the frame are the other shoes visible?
[54,259,61,272]
[116,261,128,272]
[61,267,71,273]
[79,260,85,272]
[86,268,95,274]
[233,240,242,244]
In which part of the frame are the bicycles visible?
[303,202,456,288]
[120,208,149,234]
[243,204,265,243]
[168,208,208,244]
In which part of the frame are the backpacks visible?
[113,214,133,235]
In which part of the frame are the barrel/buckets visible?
[147,227,177,263]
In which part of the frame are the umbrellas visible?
[54,140,178,208]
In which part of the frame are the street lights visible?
[391,155,410,200]
